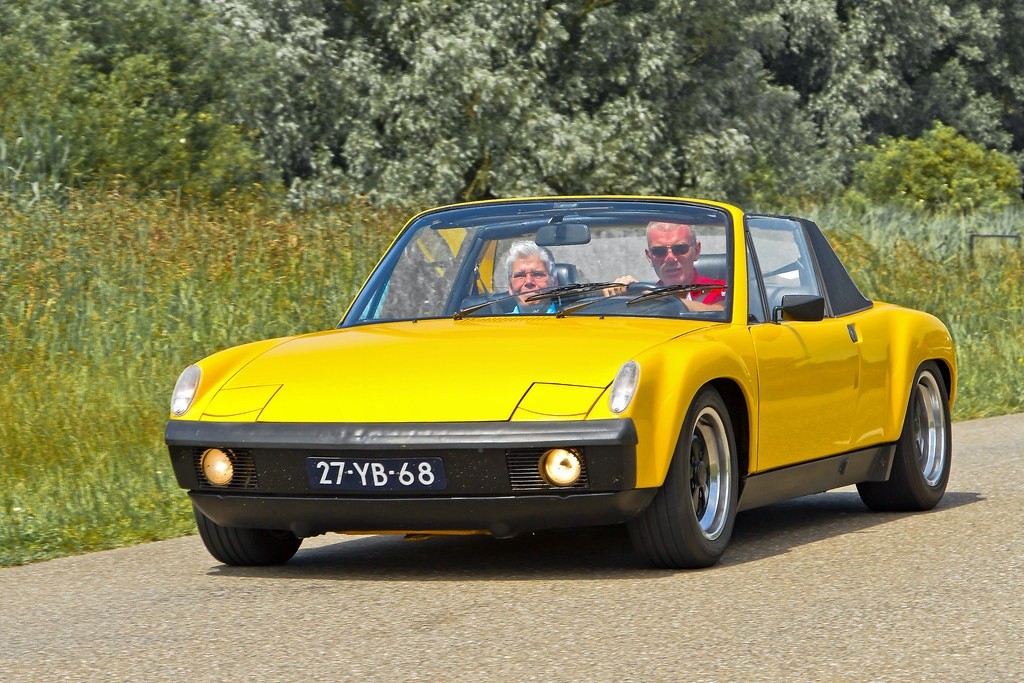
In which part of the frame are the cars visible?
[165,195,958,569]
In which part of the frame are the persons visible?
[503,240,561,316]
[602,222,728,317]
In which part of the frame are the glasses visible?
[649,241,696,255]
[509,271,549,278]
